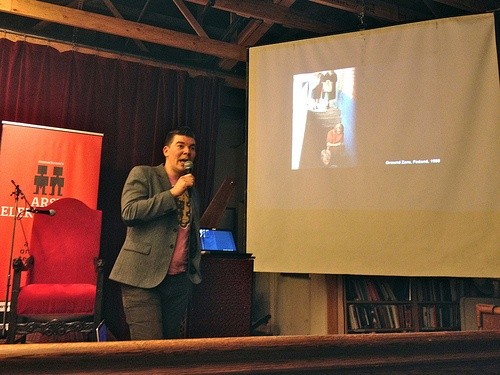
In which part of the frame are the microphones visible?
[184,161,194,198]
[27,209,56,216]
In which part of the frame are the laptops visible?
[199,227,253,256]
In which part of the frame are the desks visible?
[185,252,256,337]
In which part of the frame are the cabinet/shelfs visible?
[342,274,461,334]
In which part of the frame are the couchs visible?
[5,196,106,344]
